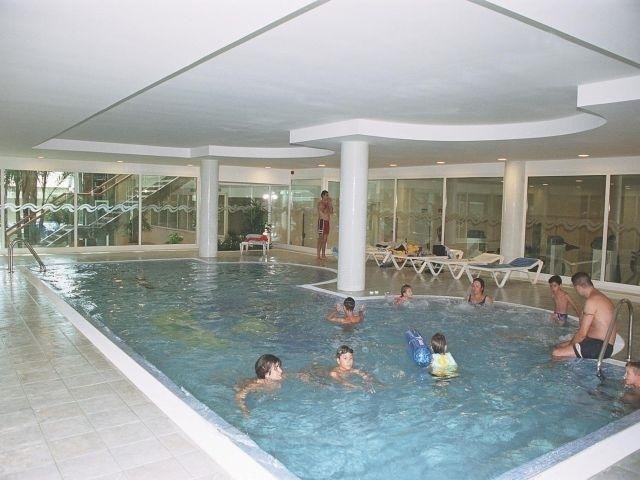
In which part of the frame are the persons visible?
[466,278,493,310]
[229,354,311,419]
[620,362,640,406]
[392,284,416,306]
[316,190,334,261]
[327,296,365,326]
[549,274,579,328]
[423,334,459,381]
[328,344,383,394]
[534,271,618,369]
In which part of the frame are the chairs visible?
[240,233,268,257]
[365,245,544,291]
